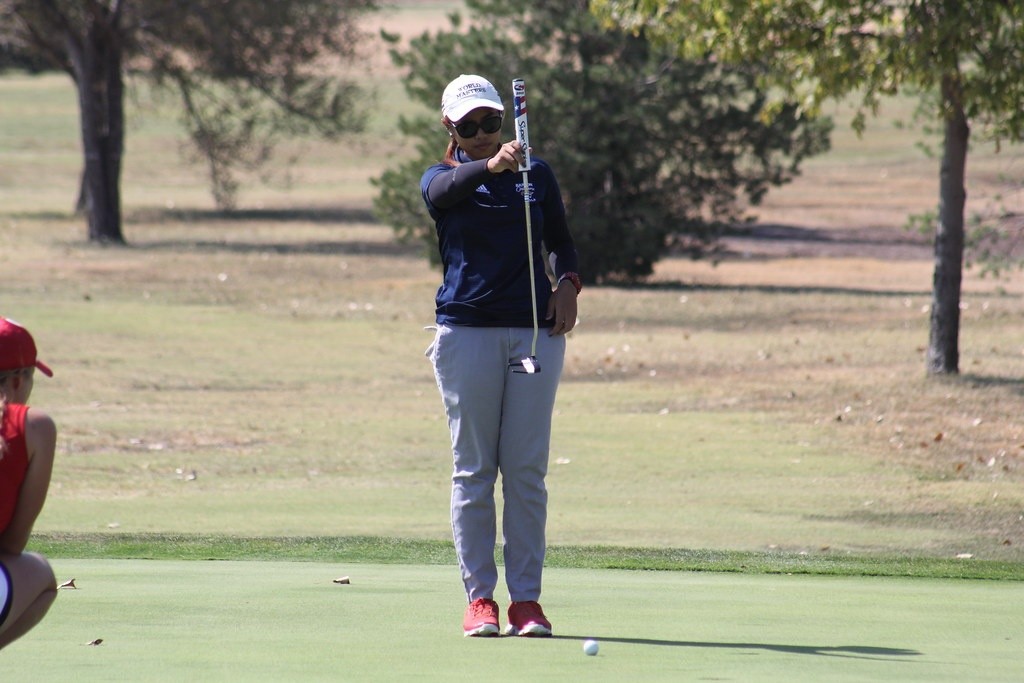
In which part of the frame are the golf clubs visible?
[509,78,543,375]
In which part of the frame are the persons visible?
[421,75,582,636]
[0,314,58,650]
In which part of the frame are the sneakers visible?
[505,601,551,636]
[463,598,501,636]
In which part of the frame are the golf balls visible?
[583,640,599,655]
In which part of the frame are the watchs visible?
[557,272,582,296]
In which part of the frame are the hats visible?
[0,317,54,378]
[441,74,504,122]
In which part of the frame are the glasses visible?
[449,110,502,138]
